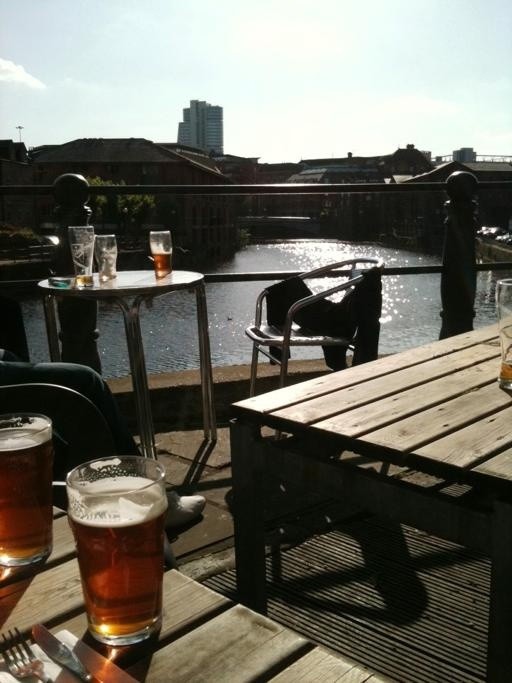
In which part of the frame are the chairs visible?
[0,382,116,463]
[246,256,385,442]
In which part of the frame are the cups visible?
[150,229,174,280]
[0,411,53,568]
[67,454,170,648]
[495,278,512,389]
[93,233,118,282]
[67,225,95,288]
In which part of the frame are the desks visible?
[37,270,218,461]
[1,504,386,683]
[229,321,511,680]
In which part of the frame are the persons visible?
[0,360,207,529]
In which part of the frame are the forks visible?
[0,626,53,683]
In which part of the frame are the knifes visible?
[31,623,101,683]
